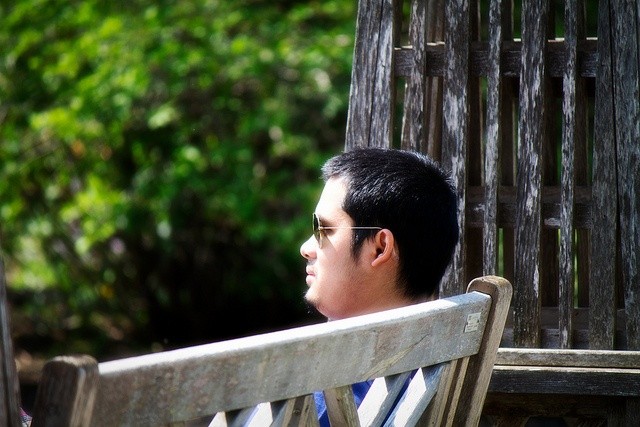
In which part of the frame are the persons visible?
[208,149,459,427]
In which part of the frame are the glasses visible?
[313,212,382,249]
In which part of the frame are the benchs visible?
[24,273,515,425]
